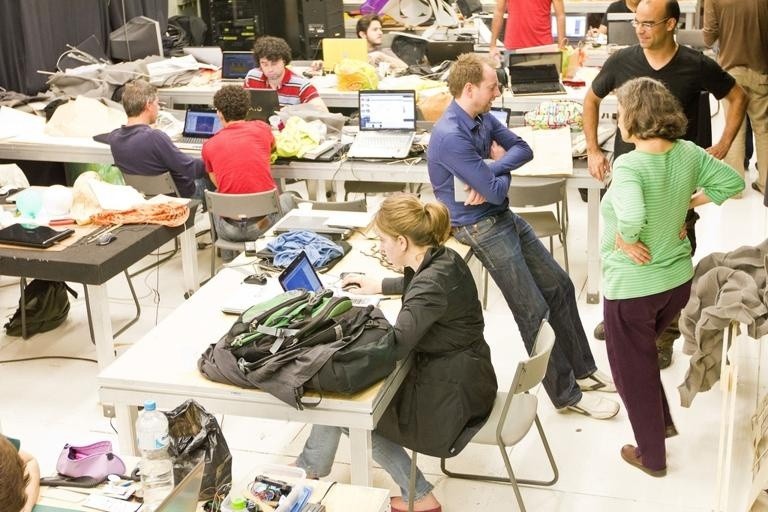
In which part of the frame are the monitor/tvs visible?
[107,15,164,61]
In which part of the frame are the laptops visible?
[489,106,511,129]
[509,52,567,97]
[322,38,367,75]
[607,20,640,47]
[244,88,281,125]
[221,51,262,87]
[347,90,416,161]
[424,41,474,67]
[174,108,224,150]
[272,214,355,241]
[551,12,588,46]
[0,222,75,249]
[279,250,380,315]
[608,13,634,20]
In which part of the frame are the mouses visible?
[96,233,117,245]
[342,283,361,293]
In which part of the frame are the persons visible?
[103,77,214,209]
[348,15,406,74]
[240,34,331,120]
[201,84,278,267]
[427,55,621,421]
[582,0,745,372]
[287,192,497,512]
[700,1,768,201]
[490,0,567,50]
[0,428,43,512]
[599,76,745,476]
[598,1,647,38]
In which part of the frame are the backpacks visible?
[196,287,408,412]
[2,274,71,339]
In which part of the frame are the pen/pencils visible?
[87,224,124,243]
[86,224,113,240]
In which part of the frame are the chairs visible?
[407,317,559,512]
[310,198,368,213]
[481,175,569,312]
[202,186,285,281]
[117,169,182,207]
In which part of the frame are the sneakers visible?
[594,317,608,341]
[654,338,672,369]
[574,371,616,390]
[565,392,621,420]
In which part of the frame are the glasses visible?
[627,16,669,31]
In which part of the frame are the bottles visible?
[135,400,175,509]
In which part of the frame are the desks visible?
[96,205,473,484]
[0,196,200,372]
[0,127,574,305]
[75,67,615,121]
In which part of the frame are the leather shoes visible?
[620,445,668,478]
[662,424,677,439]
[390,497,441,510]
